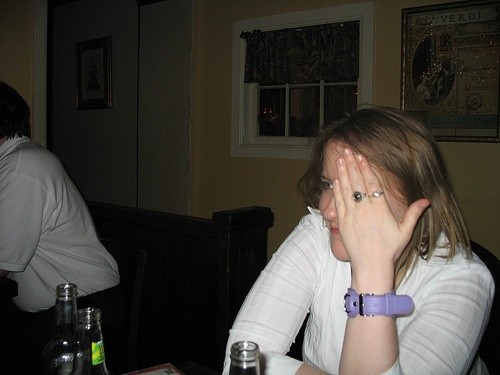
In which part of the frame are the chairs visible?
[100,239,148,375]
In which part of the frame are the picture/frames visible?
[75,35,112,111]
[401,0,500,144]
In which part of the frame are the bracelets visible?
[344,287,414,318]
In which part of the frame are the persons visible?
[1,79,136,371]
[221,107,496,375]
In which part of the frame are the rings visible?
[370,190,384,197]
[352,191,368,203]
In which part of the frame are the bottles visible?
[69,307,109,375]
[229,341,260,375]
[41,283,77,375]
